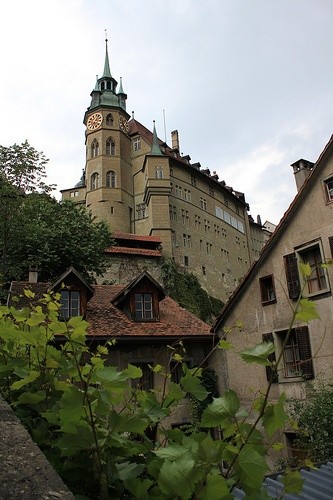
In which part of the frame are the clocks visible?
[87,113,103,130]
